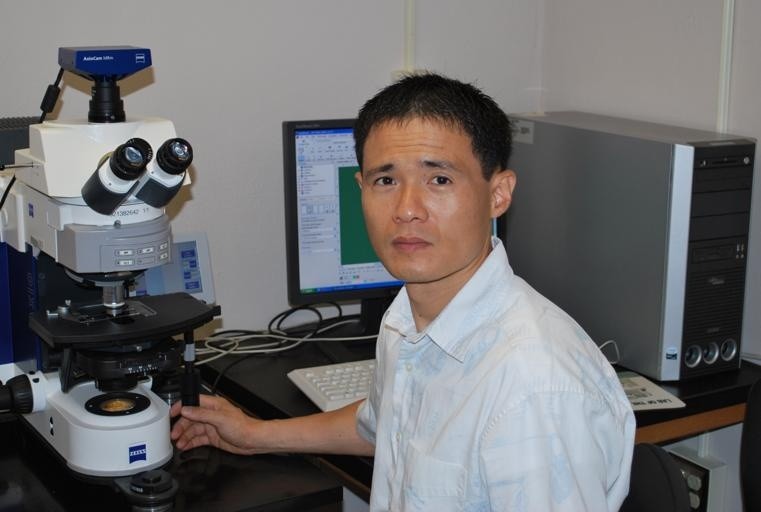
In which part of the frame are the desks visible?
[171,312,760,512]
[2,357,345,512]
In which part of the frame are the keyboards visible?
[285,358,377,413]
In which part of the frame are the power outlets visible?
[666,447,728,512]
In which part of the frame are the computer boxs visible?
[496,111,756,382]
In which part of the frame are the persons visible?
[168,71,639,512]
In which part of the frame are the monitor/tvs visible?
[281,118,509,364]
